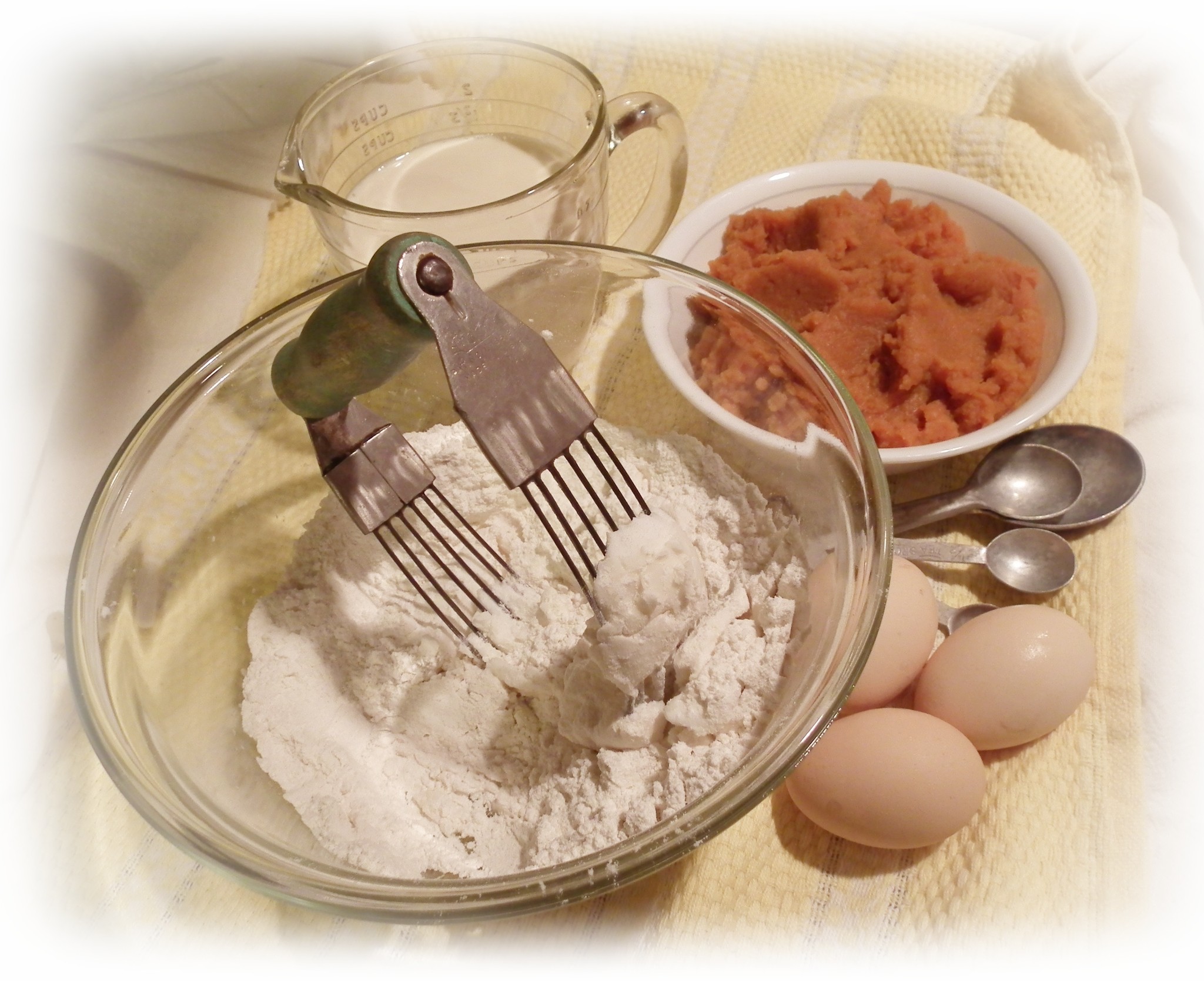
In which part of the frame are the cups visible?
[274,31,689,382]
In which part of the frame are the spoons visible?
[888,423,1151,536]
[879,442,1085,538]
[890,528,1077,595]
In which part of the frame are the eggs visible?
[796,543,939,714]
[914,604,1096,750]
[784,707,988,849]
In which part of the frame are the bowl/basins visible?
[64,236,899,930]
[634,154,1097,481]
